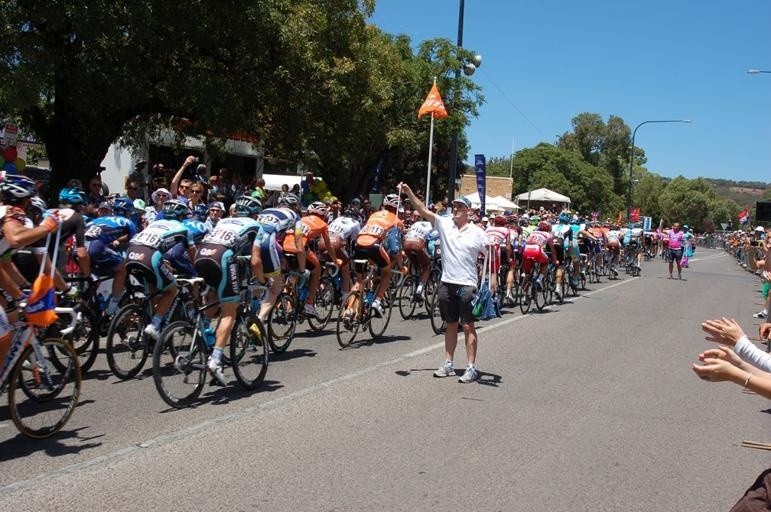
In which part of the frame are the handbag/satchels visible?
[472,283,502,321]
[679,256,690,269]
[25,272,57,326]
[383,228,402,255]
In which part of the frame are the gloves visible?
[302,270,311,279]
[45,209,67,232]
[260,278,273,287]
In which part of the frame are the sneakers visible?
[610,266,618,274]
[371,299,383,318]
[505,293,517,300]
[554,289,563,300]
[416,288,424,301]
[752,312,768,319]
[579,271,586,280]
[536,279,543,291]
[342,310,355,329]
[23,360,46,373]
[302,304,318,316]
[144,323,160,339]
[433,362,455,377]
[458,368,477,383]
[668,274,680,280]
[207,356,227,386]
[250,323,264,346]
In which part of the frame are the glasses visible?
[191,190,200,193]
[180,186,189,189]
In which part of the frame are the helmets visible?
[0,175,47,215]
[278,191,299,208]
[113,196,262,217]
[383,194,402,208]
[58,187,82,202]
[307,201,328,216]
[470,215,552,231]
[559,210,642,230]
[342,204,357,215]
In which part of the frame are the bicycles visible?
[152,276,269,412]
[2,302,83,440]
[1,232,669,402]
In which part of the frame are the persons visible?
[1,155,447,387]
[701,223,771,372]
[692,345,771,401]
[468,208,696,304]
[394,183,499,384]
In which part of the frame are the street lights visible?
[447,55,482,206]
[627,118,692,222]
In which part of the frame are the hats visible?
[451,198,472,208]
[755,226,764,232]
[151,187,173,204]
[733,229,745,235]
[683,225,688,228]
[135,158,146,166]
[98,201,112,211]
[352,198,360,202]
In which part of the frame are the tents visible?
[516,187,571,211]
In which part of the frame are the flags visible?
[737,208,750,224]
[418,85,449,121]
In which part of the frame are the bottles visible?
[299,288,307,302]
[365,292,374,303]
[204,322,216,347]
[203,312,211,324]
[252,295,259,311]
[335,276,345,289]
[98,292,105,310]
[109,297,117,313]
